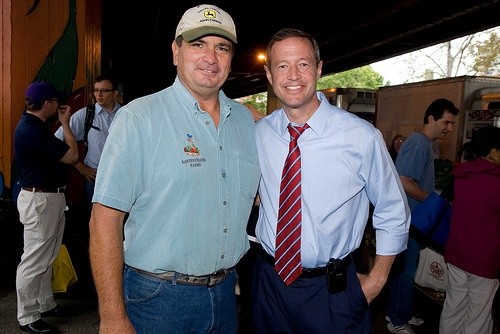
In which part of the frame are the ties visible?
[274,123,310,287]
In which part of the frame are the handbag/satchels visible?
[409,181,454,255]
[51,245,78,293]
[414,247,448,293]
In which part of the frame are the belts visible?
[265,254,351,279]
[23,185,67,193]
[131,268,227,287]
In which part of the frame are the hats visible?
[175,4,237,44]
[25,82,63,104]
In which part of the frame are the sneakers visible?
[385,315,424,326]
[387,321,416,334]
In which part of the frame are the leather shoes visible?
[40,306,80,317]
[19,319,57,334]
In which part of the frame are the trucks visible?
[315,87,376,126]
[374,75,500,190]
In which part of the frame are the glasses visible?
[93,88,114,94]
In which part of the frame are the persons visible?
[89,5,260,334]
[53,75,123,313]
[251,29,411,334]
[11,83,78,334]
[384,98,500,334]
